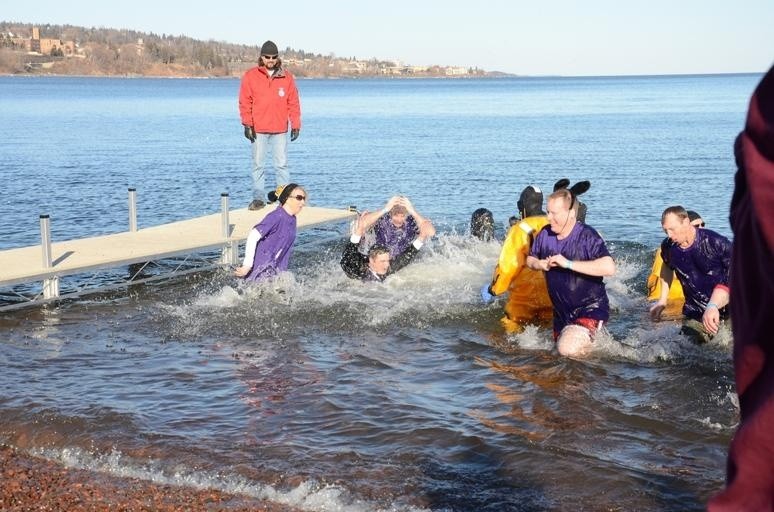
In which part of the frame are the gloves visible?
[291,129,299,141]
[245,126,257,143]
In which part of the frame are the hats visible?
[261,41,278,55]
[517,184,544,213]
[274,182,298,204]
[687,211,701,222]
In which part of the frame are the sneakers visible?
[248,200,266,211]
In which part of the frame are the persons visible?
[232,183,306,282]
[471,185,616,361]
[236,40,300,211]
[359,194,435,257]
[338,211,430,285]
[645,205,732,346]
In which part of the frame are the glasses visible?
[263,55,278,59]
[288,194,305,200]
[695,223,705,227]
